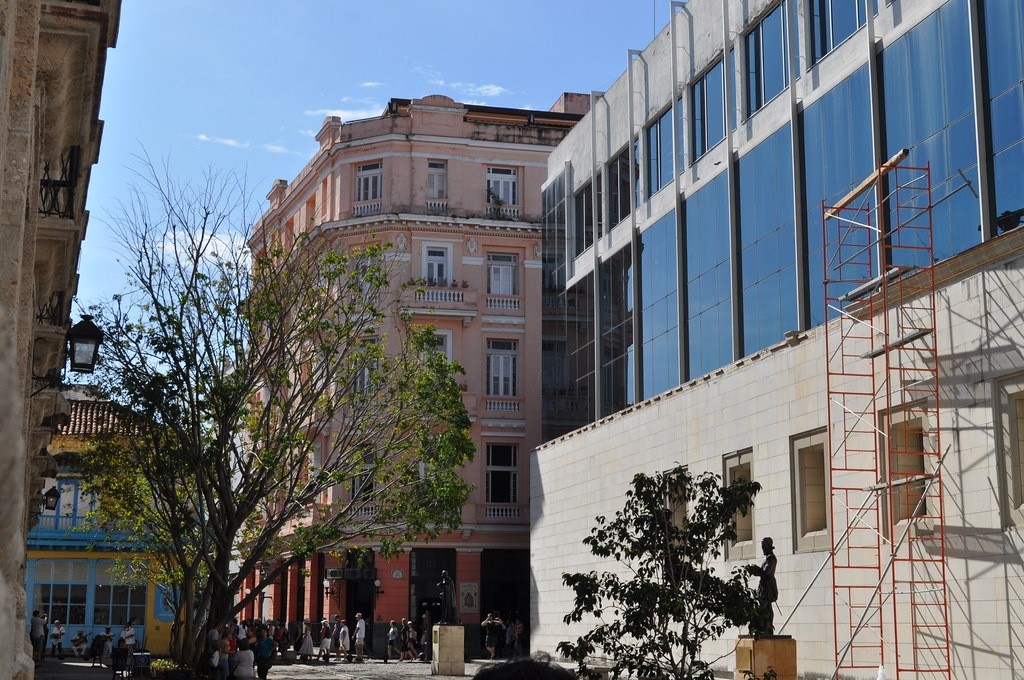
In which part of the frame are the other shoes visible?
[490,655,494,660]
[399,659,403,661]
[333,657,341,661]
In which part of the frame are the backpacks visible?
[322,626,332,639]
[210,650,219,667]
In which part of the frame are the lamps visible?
[40,485,61,510]
[260,586,273,601]
[374,579,385,599]
[245,588,258,601]
[66,314,106,373]
[323,580,337,599]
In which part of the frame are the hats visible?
[355,613,361,617]
[78,629,83,633]
[304,620,310,623]
[33,610,41,616]
[321,620,329,625]
[264,619,272,624]
[55,620,60,624]
[407,621,412,624]
[104,625,112,627]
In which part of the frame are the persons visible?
[408,621,419,659]
[233,618,290,661]
[51,620,66,657]
[316,620,331,661]
[320,618,327,633]
[387,620,400,658]
[30,610,49,660]
[298,618,313,659]
[745,537,778,635]
[99,622,135,666]
[398,618,413,661]
[71,630,87,656]
[481,608,524,660]
[207,621,279,680]
[420,611,432,659]
[472,658,579,680]
[331,614,341,661]
[339,620,350,664]
[352,613,365,661]
[437,570,459,624]
[293,619,303,655]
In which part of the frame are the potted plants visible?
[439,278,448,287]
[419,276,427,286]
[461,279,468,288]
[452,279,459,287]
[428,278,438,287]
[407,278,416,285]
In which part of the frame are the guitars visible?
[101,634,116,641]
[73,632,93,646]
